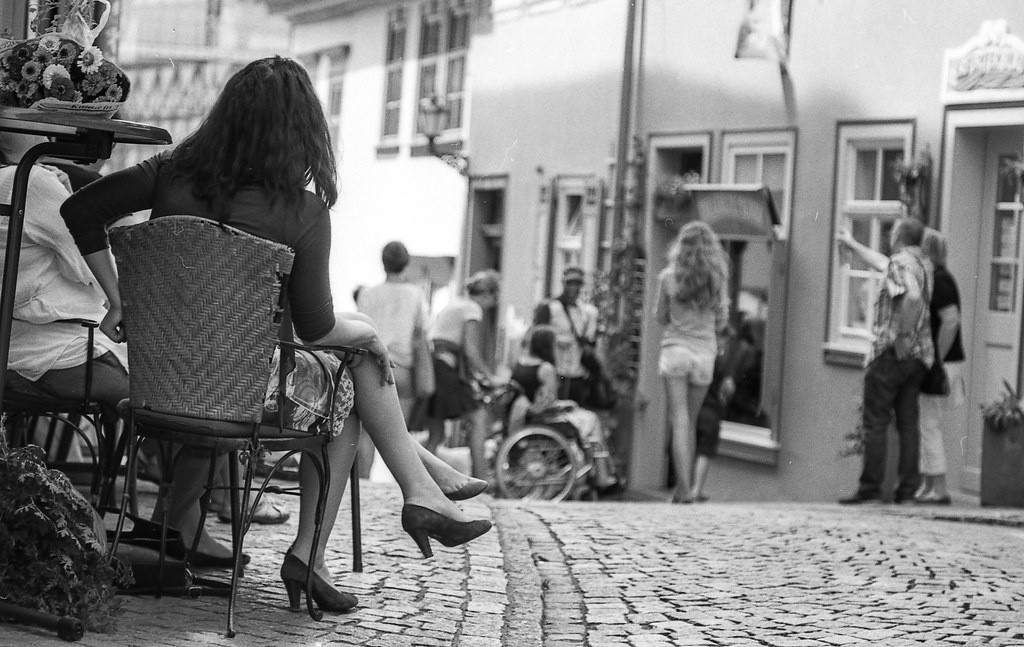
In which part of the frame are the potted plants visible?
[976,374,1024,508]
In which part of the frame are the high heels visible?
[402,504,492,559]
[281,555,358,612]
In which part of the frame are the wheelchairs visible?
[481,379,626,504]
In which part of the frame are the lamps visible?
[417,94,469,177]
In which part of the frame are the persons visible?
[690,327,736,500]
[510,328,618,489]
[651,221,732,504]
[836,215,967,503]
[59,54,500,614]
[519,263,599,398]
[0,149,250,566]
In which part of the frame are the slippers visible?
[218,498,290,523]
[209,495,225,512]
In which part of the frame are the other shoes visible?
[837,490,880,505]
[443,475,489,502]
[913,484,948,503]
[893,493,914,506]
[184,548,250,567]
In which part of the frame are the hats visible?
[564,267,584,282]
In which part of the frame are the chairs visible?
[97,213,367,637]
[11,310,138,530]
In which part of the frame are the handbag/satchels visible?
[920,351,948,394]
[582,351,600,373]
[412,304,435,397]
